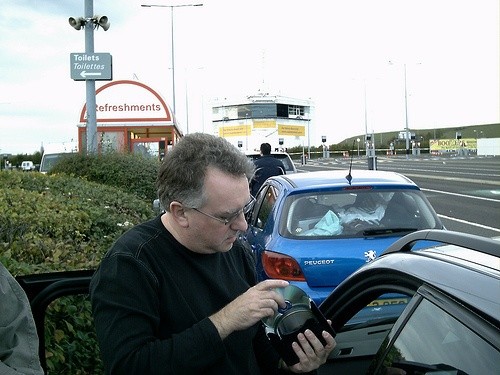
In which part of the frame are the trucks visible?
[38,142,79,177]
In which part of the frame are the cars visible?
[239,168,449,331]
[244,148,298,176]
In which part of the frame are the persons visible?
[91,132,337,375]
[248,143,285,197]
[0,264,44,374]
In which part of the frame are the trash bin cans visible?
[301,155,306,165]
[367,156,377,170]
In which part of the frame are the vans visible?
[21,161,36,171]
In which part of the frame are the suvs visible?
[278,229,500,375]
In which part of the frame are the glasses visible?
[192,194,256,225]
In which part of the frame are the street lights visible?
[389,61,423,152]
[141,3,203,116]
